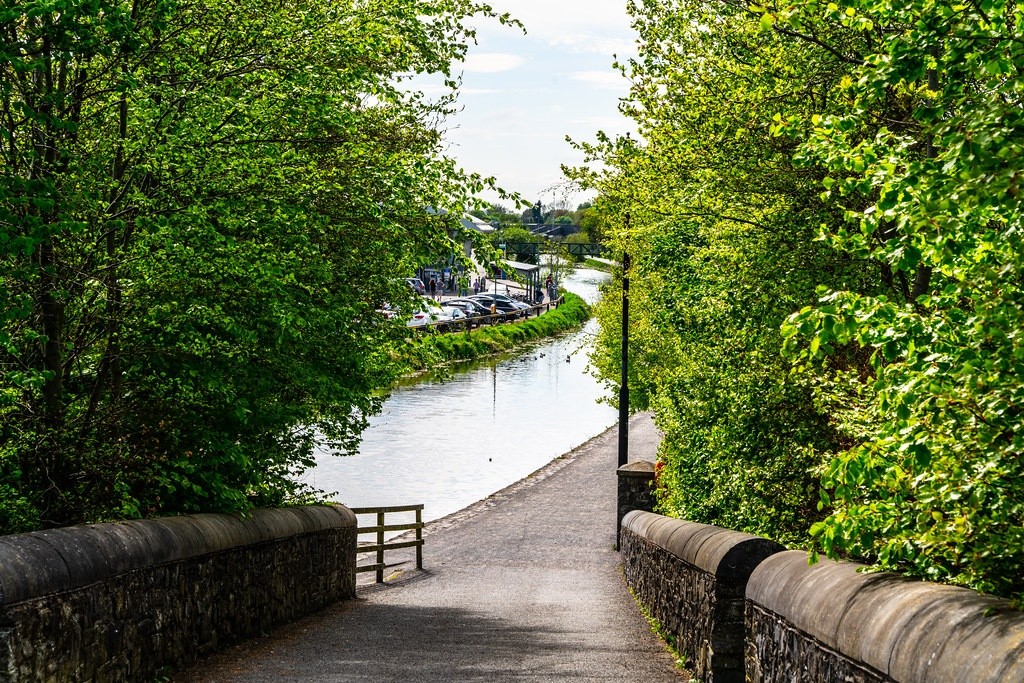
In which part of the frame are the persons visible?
[465,302,473,310]
[481,278,485,291]
[473,279,480,295]
[536,290,544,304]
[435,278,444,302]
[546,273,553,294]
[429,280,436,301]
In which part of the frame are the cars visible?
[375,276,534,333]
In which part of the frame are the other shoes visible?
[550,303,556,306]
[436,298,440,302]
